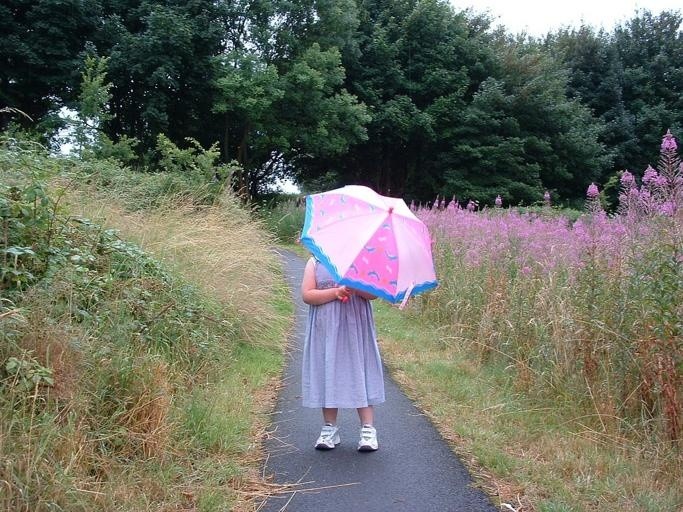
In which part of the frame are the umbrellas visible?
[300,184,441,302]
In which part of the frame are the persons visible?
[300,253,386,453]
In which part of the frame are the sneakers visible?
[357,423,379,451]
[314,423,341,449]
